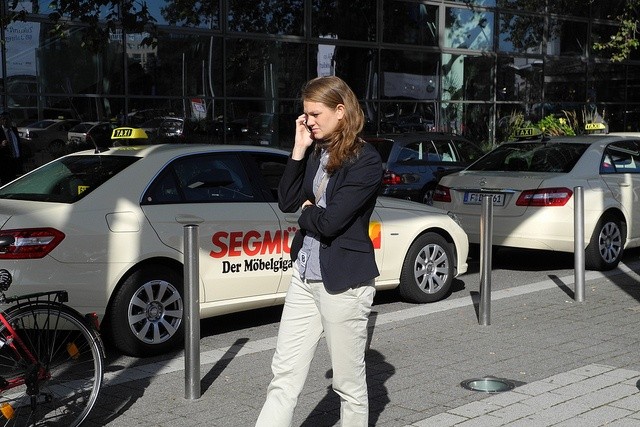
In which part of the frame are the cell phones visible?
[303,120,312,132]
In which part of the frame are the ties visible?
[7,129,16,156]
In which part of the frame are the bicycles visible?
[0,270,104,427]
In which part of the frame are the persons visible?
[254,75,384,427]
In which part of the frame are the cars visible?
[189,115,245,142]
[233,113,295,151]
[66,120,118,152]
[15,116,80,147]
[435,123,640,270]
[365,132,491,205]
[132,115,196,143]
[0,126,471,358]
